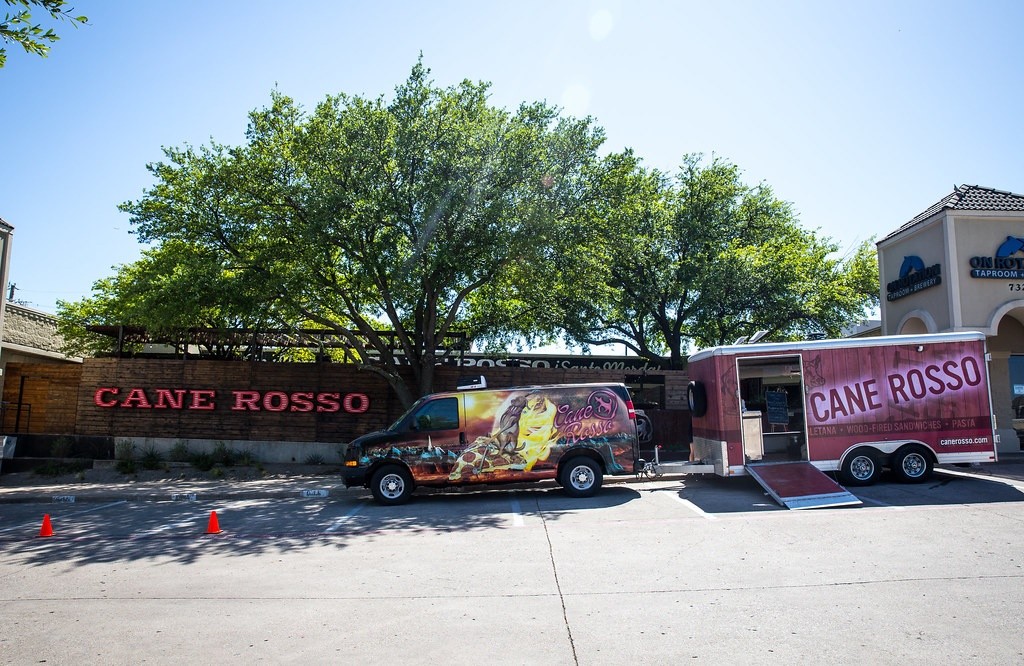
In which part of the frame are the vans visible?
[339,377,647,507]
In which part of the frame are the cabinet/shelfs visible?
[742,410,764,461]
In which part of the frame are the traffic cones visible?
[34,514,56,537]
[202,509,224,534]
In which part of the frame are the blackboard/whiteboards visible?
[765,391,790,426]
